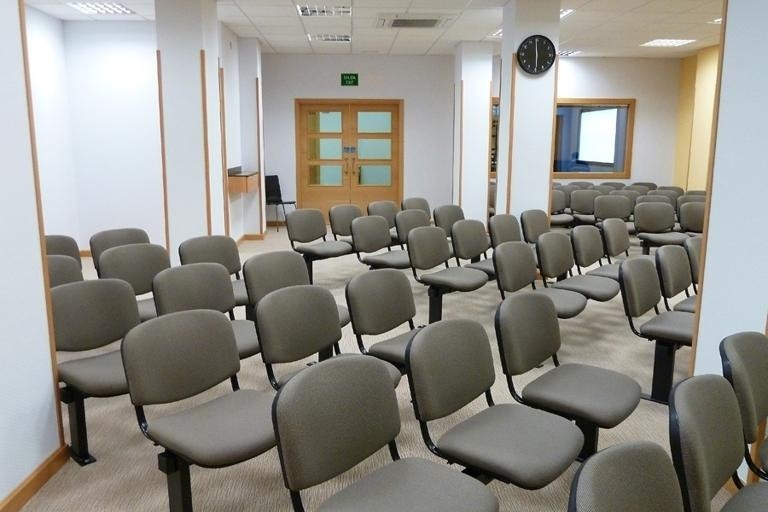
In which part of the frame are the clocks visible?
[517,34,556,74]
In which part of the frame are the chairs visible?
[264,174,298,233]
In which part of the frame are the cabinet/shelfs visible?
[488,96,499,178]
